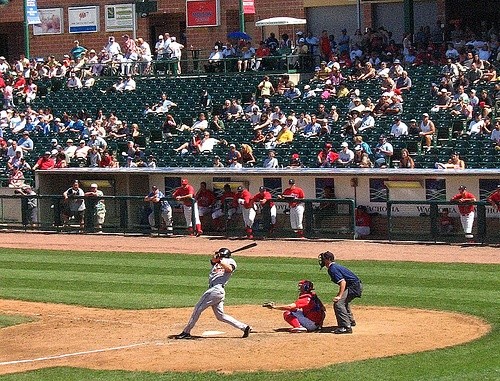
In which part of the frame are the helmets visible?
[298,279,315,290]
[215,247,231,257]
[318,250,334,270]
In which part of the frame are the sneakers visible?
[351,320,356,325]
[290,326,307,332]
[242,325,251,337]
[175,332,191,338]
[334,325,352,333]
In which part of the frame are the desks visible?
[187,48,205,73]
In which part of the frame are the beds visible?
[320,42,500,168]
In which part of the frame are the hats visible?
[23,184,30,189]
[237,185,245,191]
[259,185,266,190]
[289,179,295,183]
[182,177,189,184]
[72,179,79,183]
[90,183,98,187]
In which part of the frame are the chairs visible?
[91,75,320,167]
[207,45,317,74]
[1,71,90,190]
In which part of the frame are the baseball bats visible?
[221,243,257,258]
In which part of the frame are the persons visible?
[69,115,87,140]
[110,51,124,76]
[143,102,154,116]
[486,184,500,213]
[151,93,178,112]
[439,76,454,96]
[413,44,446,66]
[483,66,497,83]
[450,102,474,119]
[304,114,321,135]
[252,130,265,148]
[430,88,451,113]
[352,27,393,63]
[102,151,113,168]
[295,112,308,130]
[236,41,256,74]
[135,159,148,167]
[468,113,484,135]
[133,144,145,157]
[390,115,409,138]
[221,99,234,119]
[91,49,113,78]
[337,141,353,167]
[9,110,21,133]
[125,156,138,167]
[51,138,63,150]
[88,147,101,167]
[251,186,277,233]
[395,70,412,94]
[195,182,217,224]
[271,105,283,120]
[263,99,272,113]
[280,33,292,50]
[277,111,286,124]
[162,114,178,140]
[387,39,400,54]
[77,110,87,118]
[64,116,74,130]
[266,107,274,119]
[210,112,225,132]
[202,41,226,75]
[374,62,390,79]
[355,62,375,81]
[338,110,361,139]
[212,155,225,167]
[233,185,258,241]
[427,82,440,97]
[250,107,262,124]
[84,74,95,88]
[96,108,106,120]
[50,150,59,164]
[450,97,467,112]
[230,100,244,120]
[253,113,273,132]
[154,100,169,114]
[491,83,500,100]
[258,77,276,102]
[85,183,106,234]
[264,32,279,51]
[277,76,294,95]
[290,153,308,168]
[263,279,327,333]
[354,144,374,169]
[124,140,136,156]
[469,89,479,106]
[119,48,138,77]
[290,38,308,70]
[87,130,108,154]
[228,157,243,169]
[79,49,99,80]
[403,48,416,68]
[133,38,144,59]
[24,116,35,131]
[320,30,334,60]
[62,111,69,123]
[61,54,74,75]
[240,144,255,167]
[172,178,203,237]
[455,72,469,92]
[0,52,65,107]
[122,34,139,62]
[64,139,77,168]
[475,42,492,61]
[301,84,316,100]
[329,105,338,122]
[109,110,122,126]
[263,131,278,150]
[35,109,45,117]
[409,118,421,134]
[337,29,350,52]
[318,61,332,83]
[159,32,172,74]
[319,84,336,100]
[399,148,414,168]
[453,83,468,102]
[63,180,87,232]
[463,52,474,71]
[86,118,121,137]
[19,157,32,171]
[37,115,47,132]
[67,73,83,90]
[445,43,462,58]
[0,136,6,161]
[105,36,123,62]
[263,150,279,169]
[471,55,485,70]
[480,89,493,118]
[221,43,238,75]
[54,152,67,169]
[328,34,336,50]
[130,123,144,140]
[465,33,479,45]
[284,82,301,102]
[373,134,394,168]
[8,164,25,188]
[154,35,165,73]
[277,179,309,240]
[440,58,459,82]
[225,144,243,168]
[337,83,350,97]
[172,130,202,156]
[315,103,328,125]
[316,154,333,168]
[7,141,23,158]
[54,118,65,133]
[13,113,26,133]
[144,185,174,237]
[468,61,483,85]
[340,52,353,69]
[176,112,209,133]
[116,120,130,140]
[451,151,466,169]
[304,114,312,122]
[147,154,158,168]
[353,110,376,135]
[309,66,321,83]
[346,60,364,81]
[294,31,306,46]
[376,76,403,116]
[438,208,454,234]
[175,248,252,339]
[306,32,320,70]
[466,117,492,136]
[71,52,88,80]
[116,74,137,92]
[75,140,92,167]
[268,119,283,137]
[490,121,500,151]
[32,151,54,170]
[417,112,435,152]
[8,151,22,171]
[1,108,14,128]
[316,143,339,165]
[43,109,54,134]
[245,97,260,113]
[69,40,88,61]
[288,109,297,124]
[318,251,362,334]
[0,128,4,138]
[364,96,375,112]
[350,98,366,111]
[210,183,236,233]
[352,135,373,156]
[353,204,372,241]
[249,41,270,71]
[167,37,184,74]
[444,158,454,169]
[320,118,332,140]
[200,89,213,110]
[464,46,474,56]
[138,38,153,77]
[417,19,500,43]
[285,117,296,135]
[100,77,124,93]
[450,185,477,243]
[390,59,403,78]
[276,123,294,145]
[18,130,34,154]
[197,131,228,154]
[14,184,38,230]
[401,32,412,55]
[23,105,37,117]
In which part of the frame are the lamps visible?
[350,178,358,187]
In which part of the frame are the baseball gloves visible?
[262,302,273,310]
